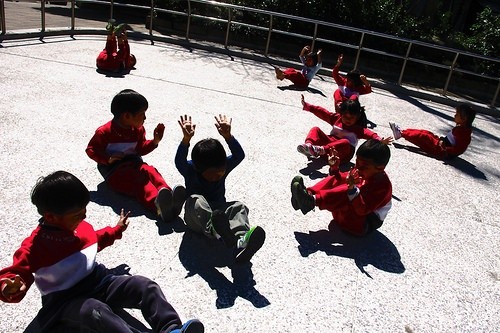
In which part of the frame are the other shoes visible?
[278,70,285,80]
[106,18,116,30]
[392,122,401,139]
[292,181,315,215]
[172,184,187,217]
[275,67,280,78]
[112,23,127,35]
[291,174,308,210]
[169,319,204,333]
[389,122,400,133]
[154,187,174,223]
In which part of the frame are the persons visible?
[291,138,392,235]
[85,89,187,222]
[174,113,267,265]
[389,104,476,160]
[297,94,394,165]
[0,171,204,333]
[333,54,371,112]
[96,19,136,74]
[276,44,322,89]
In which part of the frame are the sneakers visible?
[308,144,324,157]
[297,142,311,157]
[233,226,266,264]
[211,210,236,247]
